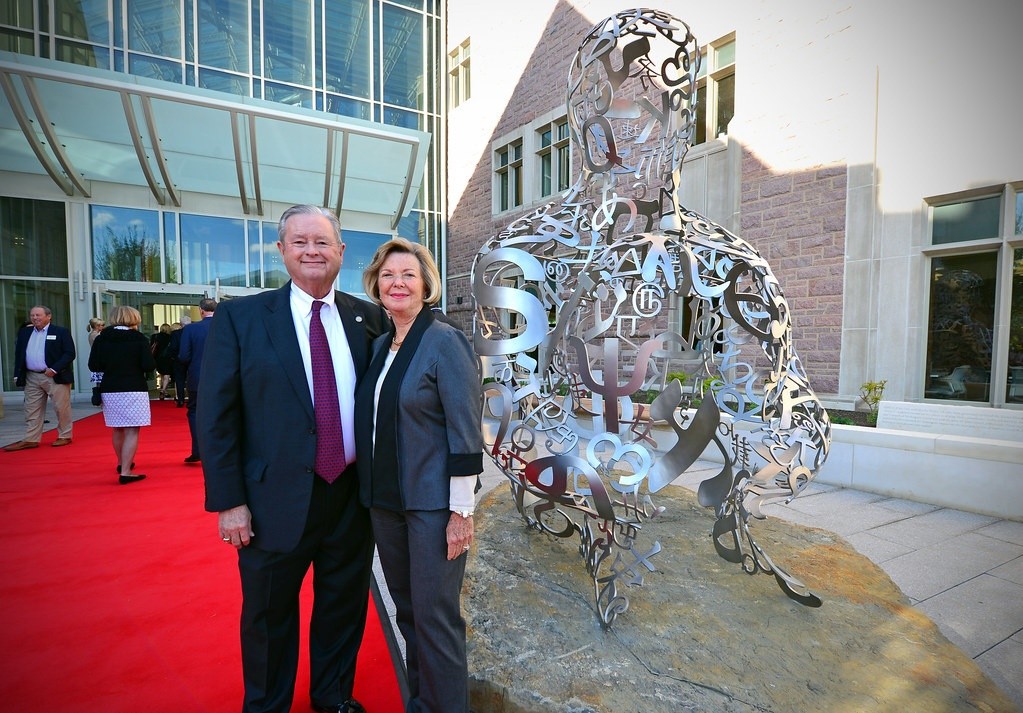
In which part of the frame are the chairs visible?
[929,365,970,400]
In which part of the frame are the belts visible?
[28,369,46,374]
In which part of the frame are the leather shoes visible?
[116,463,135,472]
[120,474,146,484]
[4,441,38,451]
[310,697,366,713]
[177,400,184,408]
[52,438,72,445]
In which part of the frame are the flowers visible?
[90,372,104,387]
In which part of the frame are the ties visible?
[309,301,347,485]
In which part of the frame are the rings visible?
[223,538,230,542]
[463,545,471,551]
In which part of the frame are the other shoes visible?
[183,455,201,462]
[159,390,165,401]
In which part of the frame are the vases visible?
[91,387,102,406]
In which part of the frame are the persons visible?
[150,316,191,407]
[175,299,218,463]
[86,318,105,408]
[88,307,154,484]
[195,203,391,713]
[5,306,76,451]
[356,238,484,713]
